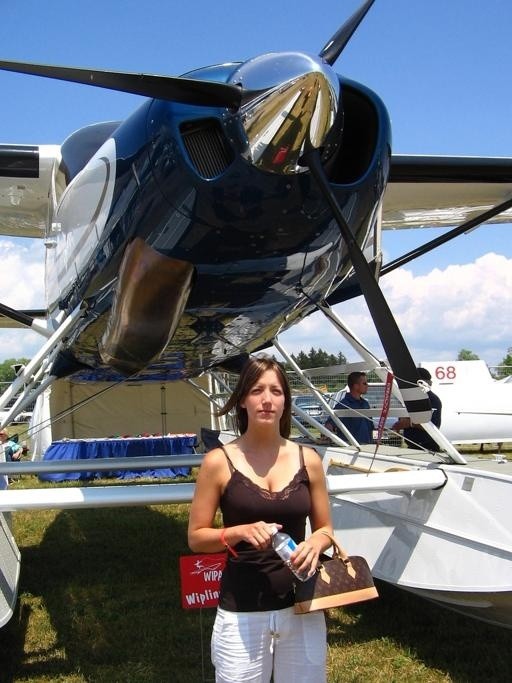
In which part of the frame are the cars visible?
[291,383,405,445]
[0,407,33,425]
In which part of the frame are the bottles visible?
[268,527,316,582]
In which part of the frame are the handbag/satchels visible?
[293,529,380,615]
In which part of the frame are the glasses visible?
[355,382,366,385]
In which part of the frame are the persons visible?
[187,362,333,683]
[0,427,23,480]
[391,367,442,452]
[322,372,375,444]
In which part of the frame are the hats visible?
[417,368,431,380]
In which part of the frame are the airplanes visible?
[1,0,511,629]
